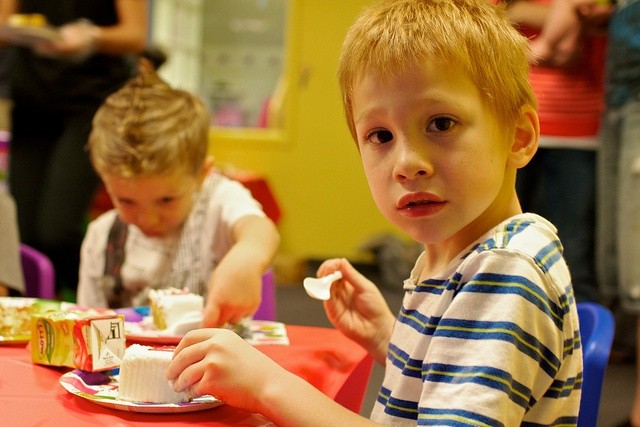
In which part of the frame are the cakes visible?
[147,286,206,334]
[116,344,195,406]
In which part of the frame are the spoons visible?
[303,270,344,301]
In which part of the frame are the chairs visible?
[574,296,616,426]
[253,275,275,324]
[21,245,56,300]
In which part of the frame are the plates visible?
[0,25,62,47]
[102,304,186,347]
[0,296,102,348]
[58,367,224,413]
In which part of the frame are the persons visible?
[489,0,620,302]
[74,61,284,328]
[580,2,640,368]
[165,0,586,427]
[1,0,152,303]
